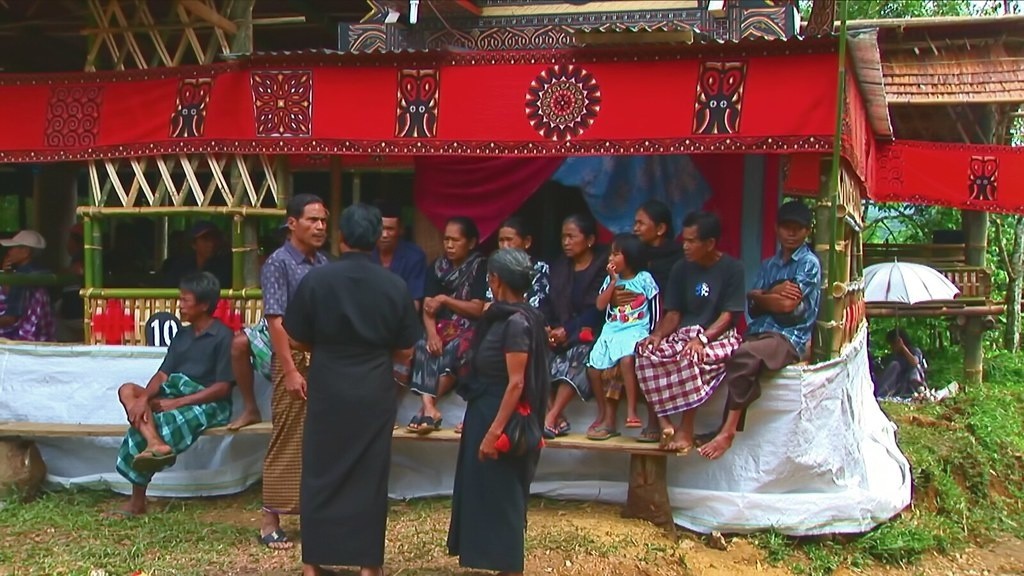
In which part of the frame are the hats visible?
[779,201,813,226]
[0,230,47,249]
[61,223,83,237]
[190,220,220,238]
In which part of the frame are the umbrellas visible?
[863,256,962,338]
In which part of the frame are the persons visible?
[697,202,822,458]
[281,203,426,576]
[101,271,235,520]
[447,249,549,576]
[0,219,236,344]
[874,328,929,403]
[368,201,746,452]
[257,193,333,551]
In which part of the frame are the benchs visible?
[0,420,696,540]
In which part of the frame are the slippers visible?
[586,427,622,439]
[259,529,295,550]
[637,427,660,442]
[131,449,177,472]
[407,416,442,435]
[99,507,146,520]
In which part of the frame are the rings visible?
[552,337,555,341]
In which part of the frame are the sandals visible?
[544,414,570,438]
[589,421,602,431]
[624,416,643,427]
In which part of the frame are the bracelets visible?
[698,334,709,346]
